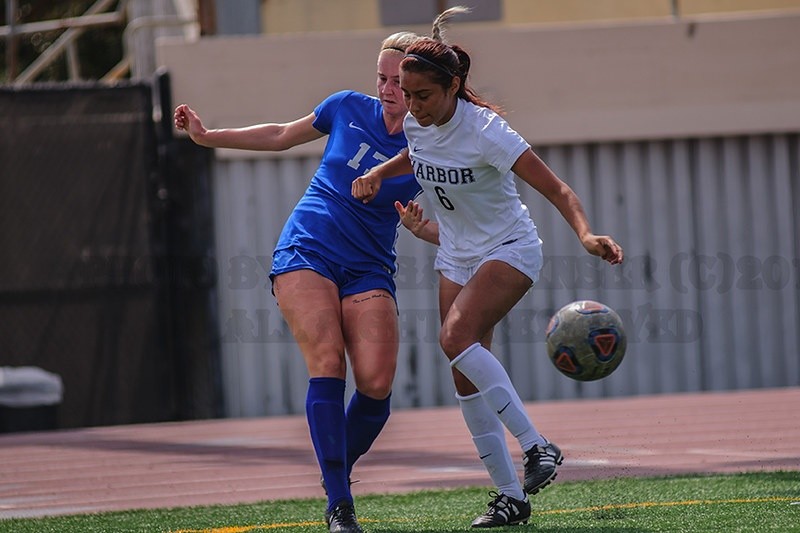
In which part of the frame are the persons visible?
[174,32,440,533]
[350,7,625,529]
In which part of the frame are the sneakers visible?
[327,500,364,533]
[319,474,361,500]
[522,435,564,494]
[471,487,532,529]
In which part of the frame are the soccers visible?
[545,300,626,383]
